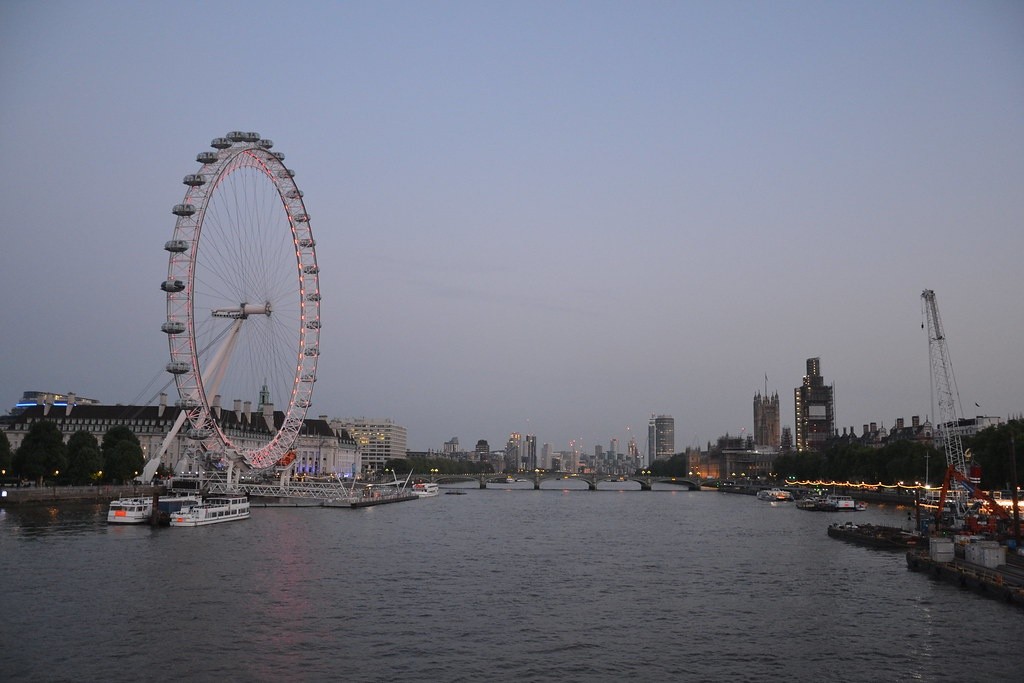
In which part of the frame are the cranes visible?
[920,288,971,519]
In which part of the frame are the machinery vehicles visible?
[931,464,1024,538]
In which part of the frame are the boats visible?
[793,496,838,512]
[757,487,793,502]
[168,497,251,527]
[106,496,203,526]
[412,483,440,499]
[825,495,855,511]
[855,501,867,510]
[826,521,932,553]
